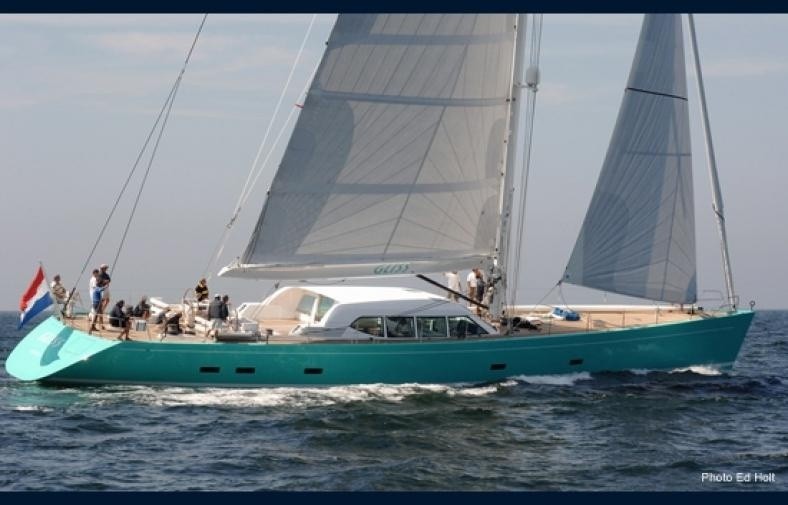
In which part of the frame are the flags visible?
[15,266,54,332]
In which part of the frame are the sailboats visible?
[3,13,761,389]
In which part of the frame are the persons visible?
[217,295,230,324]
[97,263,111,312]
[163,311,184,335]
[132,296,152,321]
[194,277,210,301]
[87,269,101,322]
[50,274,76,321]
[156,305,171,324]
[475,270,489,315]
[206,294,222,321]
[110,299,134,342]
[443,268,462,304]
[467,267,484,305]
[90,278,108,332]
